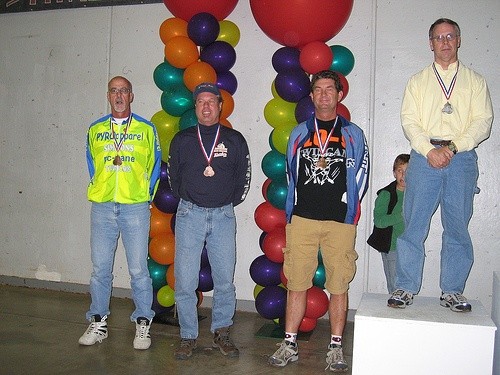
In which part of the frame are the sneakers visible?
[132,316,153,350]
[388,288,414,308]
[325,343,348,373]
[211,326,240,357]
[267,339,299,367]
[175,337,198,360]
[79,314,109,345]
[440,289,471,312]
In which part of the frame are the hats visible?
[194,83,222,102]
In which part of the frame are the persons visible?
[166,83,252,361]
[387,18,495,313]
[78,75,163,350]
[366,154,410,294]
[265,70,370,372]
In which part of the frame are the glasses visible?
[107,87,131,95]
[431,34,460,42]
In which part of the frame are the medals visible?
[203,165,215,177]
[318,158,326,167]
[113,155,122,165]
[443,102,453,113]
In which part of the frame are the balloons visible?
[139,0,241,320]
[246,0,361,333]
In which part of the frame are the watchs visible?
[447,141,458,155]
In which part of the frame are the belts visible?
[430,140,451,145]
